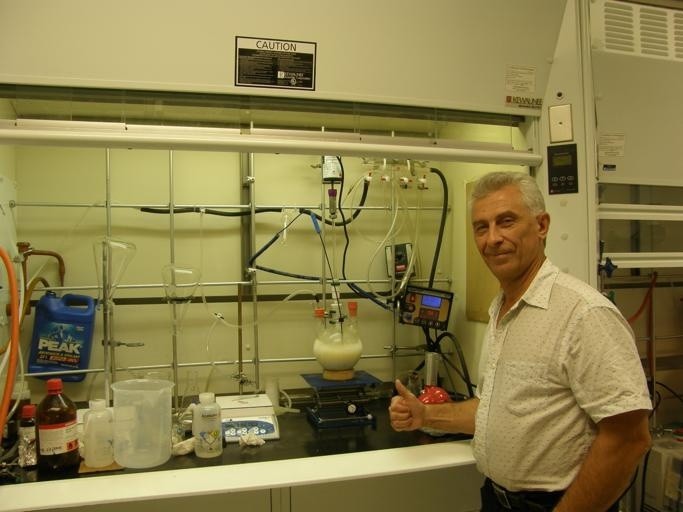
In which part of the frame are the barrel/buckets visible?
[27,291,95,382]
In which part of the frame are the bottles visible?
[6,380,31,441]
[17,402,36,473]
[312,301,365,381]
[190,391,223,460]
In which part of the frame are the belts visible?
[489,480,559,512]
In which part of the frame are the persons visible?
[387,171,654,511]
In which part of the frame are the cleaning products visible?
[193,393,222,458]
